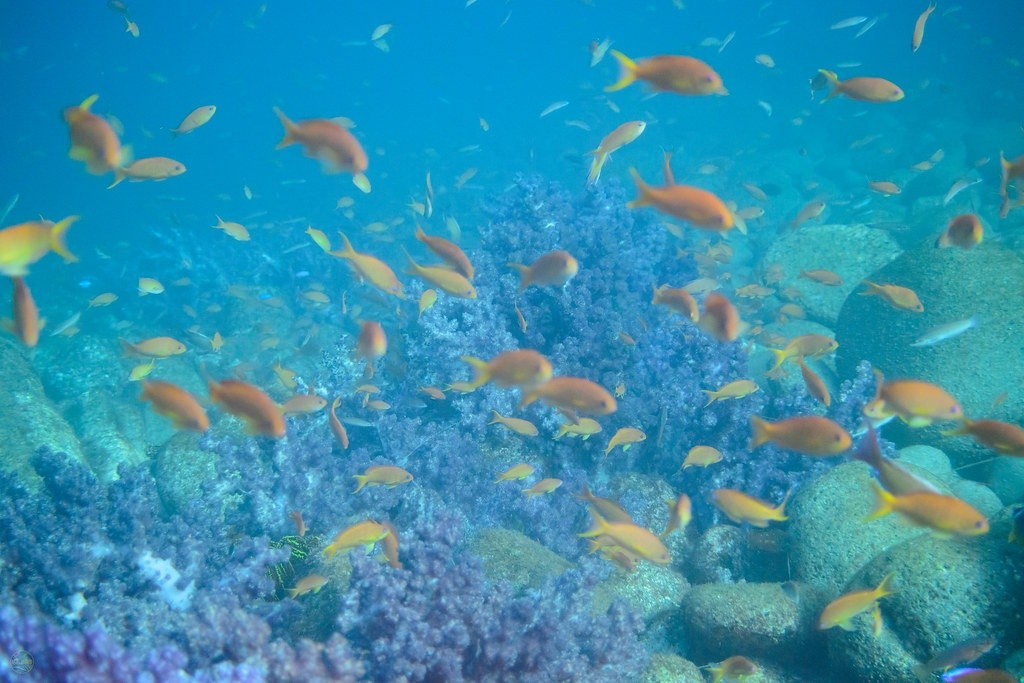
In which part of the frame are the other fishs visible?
[1,0,1024,683]
